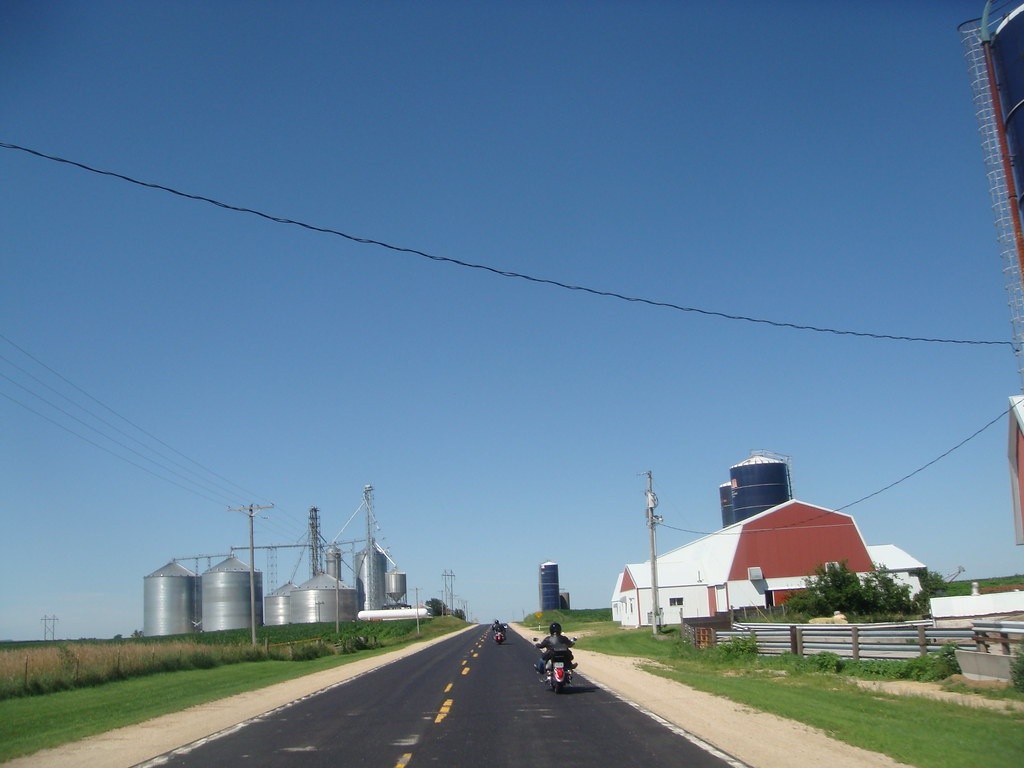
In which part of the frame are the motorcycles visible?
[532,636,578,695]
[490,625,509,643]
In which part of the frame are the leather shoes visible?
[568,663,577,670]
[536,667,544,674]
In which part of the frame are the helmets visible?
[495,620,498,623]
[550,623,561,635]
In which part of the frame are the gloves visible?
[570,638,576,644]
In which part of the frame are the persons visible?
[533,623,578,674]
[492,620,506,639]
